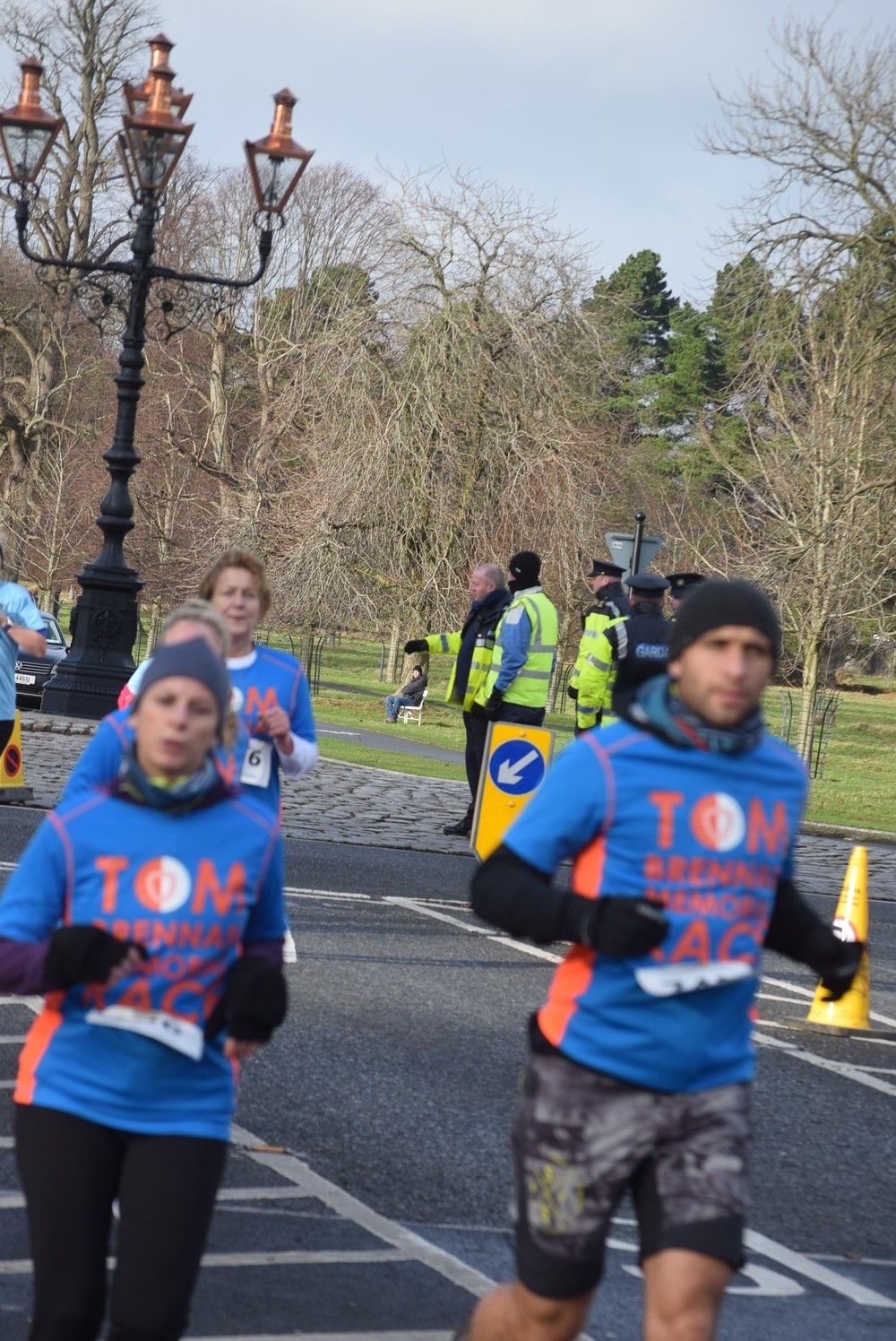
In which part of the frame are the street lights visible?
[1,31,316,714]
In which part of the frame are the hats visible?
[131,640,232,728]
[413,665,422,673]
[666,573,705,597]
[510,552,543,579]
[587,559,626,577]
[668,580,781,662]
[626,574,668,597]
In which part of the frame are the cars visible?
[15,610,71,711]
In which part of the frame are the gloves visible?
[577,897,670,960]
[470,702,485,717]
[568,686,578,699]
[404,638,429,654]
[819,942,864,1002]
[485,692,502,712]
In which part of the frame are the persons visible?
[0,545,47,758]
[404,549,707,840]
[0,551,319,1341]
[457,581,868,1341]
[384,665,425,724]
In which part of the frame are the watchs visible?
[2,616,15,631]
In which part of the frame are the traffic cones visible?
[0,715,35,800]
[779,845,896,1041]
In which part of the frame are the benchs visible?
[392,689,428,725]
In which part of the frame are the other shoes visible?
[384,718,395,724]
[444,815,472,837]
